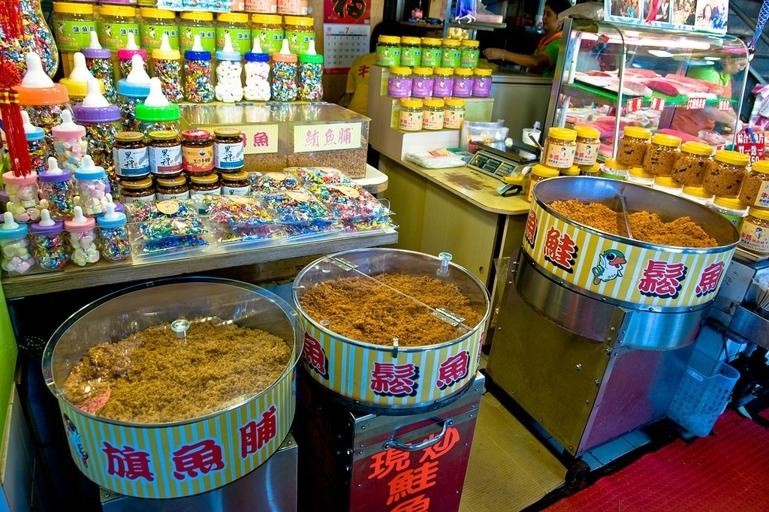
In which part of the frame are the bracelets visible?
[501,49,506,60]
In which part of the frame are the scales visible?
[467,138,540,183]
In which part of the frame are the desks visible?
[416,152,547,305]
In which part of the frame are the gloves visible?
[484,48,507,61]
[735,118,742,124]
[731,122,742,134]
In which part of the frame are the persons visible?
[611,0,641,19]
[645,0,670,23]
[674,0,695,25]
[671,36,756,136]
[345,20,403,198]
[483,0,572,78]
[697,1,728,32]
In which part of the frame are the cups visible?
[521,128,541,148]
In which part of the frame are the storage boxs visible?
[265,101,371,178]
[178,104,287,178]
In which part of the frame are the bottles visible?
[64,205,100,267]
[739,207,769,256]
[60,51,106,108]
[423,97,445,130]
[72,78,122,200]
[140,6,180,54]
[2,161,39,223]
[561,166,580,177]
[52,1,96,51]
[73,155,113,214]
[135,77,181,142]
[601,158,627,180]
[709,196,748,233]
[51,110,88,174]
[542,127,577,168]
[180,12,216,55]
[272,39,297,102]
[0,211,35,275]
[421,38,442,68]
[17,51,75,150]
[30,209,66,271]
[400,36,421,68]
[184,35,215,103]
[215,34,243,103]
[97,202,132,262]
[112,132,151,181]
[80,30,115,86]
[243,37,271,101]
[651,174,682,197]
[284,15,316,54]
[151,33,183,103]
[412,67,434,98]
[616,126,650,168]
[671,141,713,187]
[442,39,461,67]
[97,4,139,51]
[216,13,251,54]
[627,166,653,187]
[472,68,493,97]
[182,129,214,175]
[20,110,48,175]
[221,170,251,196]
[681,184,713,208]
[38,157,74,220]
[148,131,184,179]
[642,132,682,176]
[461,39,480,68]
[573,125,601,166]
[526,164,560,203]
[214,128,245,173]
[398,97,423,132]
[577,162,601,178]
[298,40,324,102]
[117,55,150,132]
[738,160,769,209]
[444,97,465,129]
[120,178,155,205]
[251,14,284,55]
[453,67,474,97]
[116,32,148,78]
[387,67,413,98]
[702,149,750,198]
[156,175,189,200]
[433,67,455,98]
[375,35,400,67]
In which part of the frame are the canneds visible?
[189,173,221,198]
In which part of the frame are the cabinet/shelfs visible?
[0,163,414,379]
[541,19,750,171]
[368,62,493,160]
[377,156,528,315]
